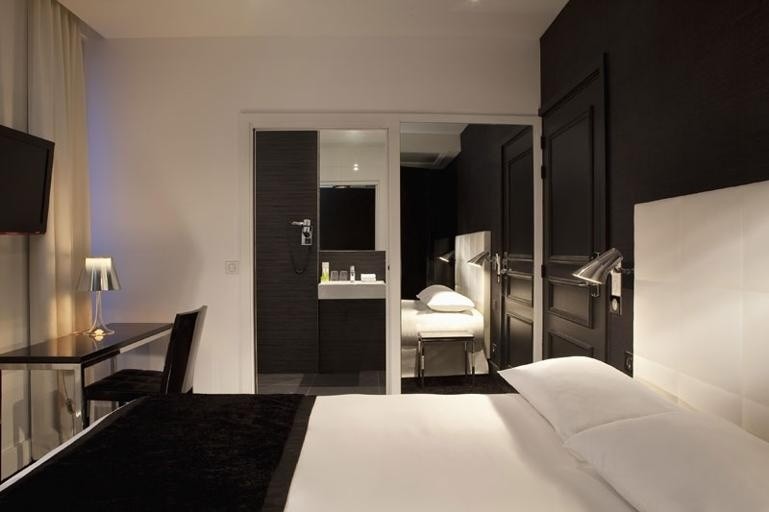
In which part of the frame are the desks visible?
[0,322,174,431]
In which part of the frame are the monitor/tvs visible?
[0,125,55,235]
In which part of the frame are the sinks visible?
[316,280,386,301]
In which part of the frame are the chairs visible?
[84,305,207,428]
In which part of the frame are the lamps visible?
[75,257,122,336]
[465,250,492,267]
[436,250,456,263]
[572,247,633,285]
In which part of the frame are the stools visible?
[416,330,476,388]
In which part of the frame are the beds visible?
[0,180,769,511]
[401,230,492,378]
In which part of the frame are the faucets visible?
[349,265,356,282]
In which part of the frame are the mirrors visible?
[399,122,533,393]
[319,184,375,251]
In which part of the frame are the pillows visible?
[497,355,678,442]
[416,284,476,312]
[563,408,769,511]
